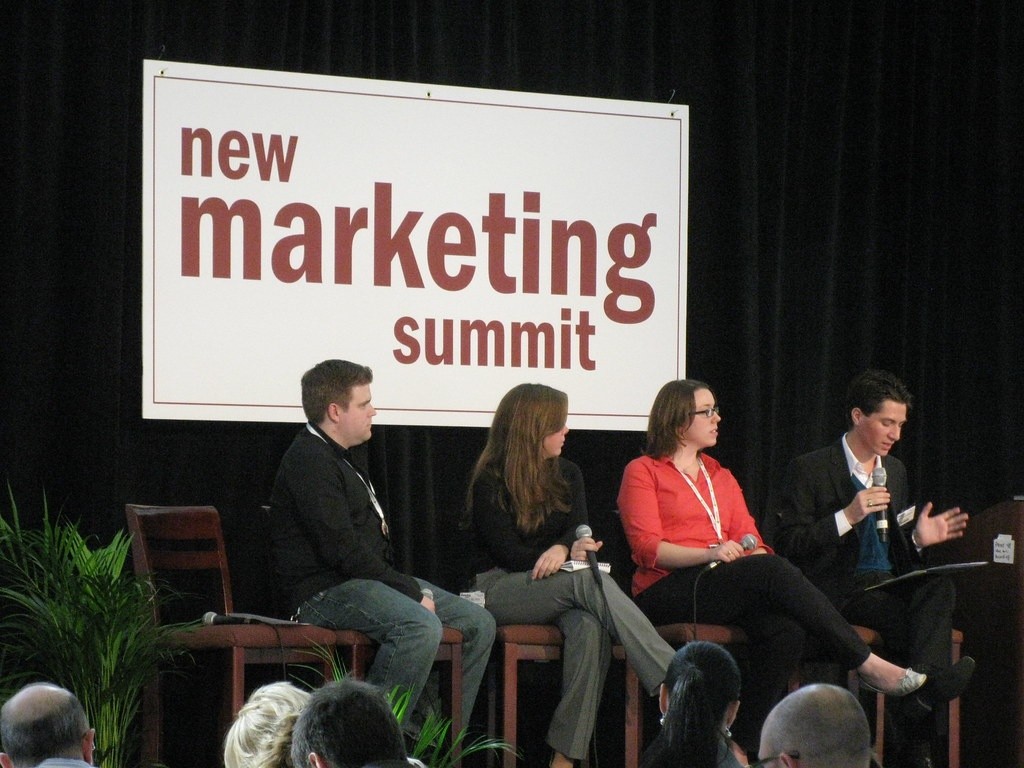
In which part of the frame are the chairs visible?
[612,510,800,693]
[776,509,965,768]
[332,628,464,768]
[127,504,336,768]
[497,626,639,768]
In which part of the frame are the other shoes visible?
[859,669,926,696]
[901,656,975,721]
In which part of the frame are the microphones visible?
[202,611,260,626]
[705,534,757,571]
[575,524,603,588]
[872,468,888,543]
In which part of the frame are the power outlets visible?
[992,535,1014,564]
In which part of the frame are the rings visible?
[729,551,734,556]
[867,499,872,508]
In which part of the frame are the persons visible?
[617,376,932,768]
[224,682,310,768]
[774,366,976,768]
[640,640,745,768]
[291,678,428,768]
[756,683,872,768]
[0,680,99,768]
[268,359,496,768]
[443,383,676,768]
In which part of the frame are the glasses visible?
[689,407,719,417]
